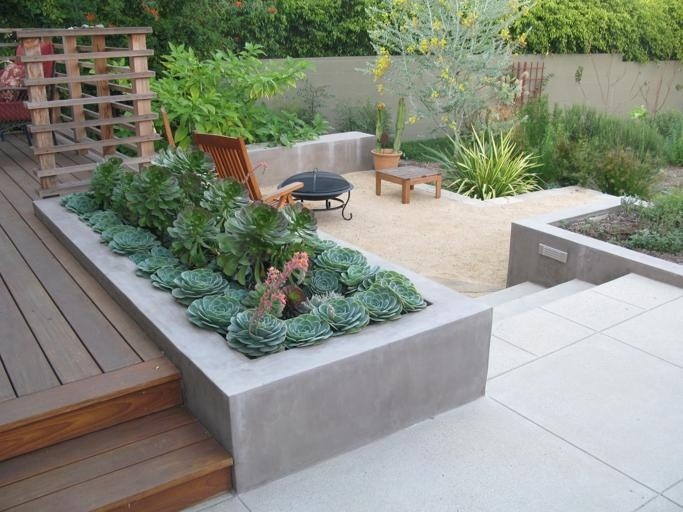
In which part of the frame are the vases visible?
[371,148,403,172]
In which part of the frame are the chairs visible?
[0,37,57,145]
[192,132,303,207]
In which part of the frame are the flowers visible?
[375,97,408,153]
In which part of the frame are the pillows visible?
[1,63,26,102]
